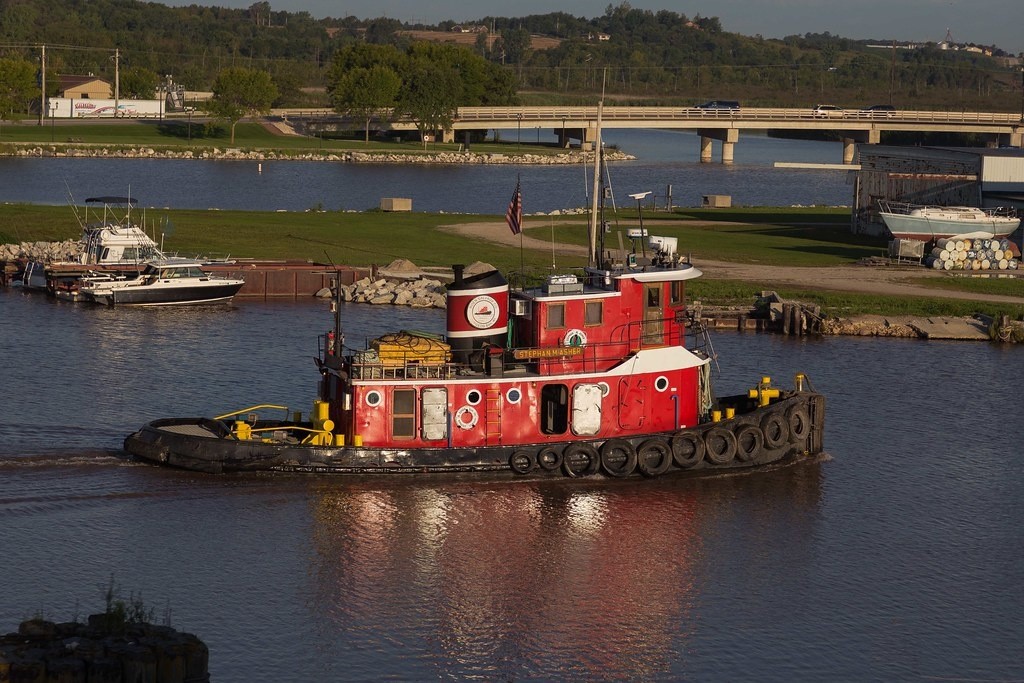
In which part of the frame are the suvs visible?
[861,105,897,118]
[813,104,849,118]
[694,100,739,110]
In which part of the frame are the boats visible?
[44,177,236,266]
[877,199,1022,243]
[123,107,826,483]
[77,234,245,307]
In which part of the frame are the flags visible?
[505,173,523,236]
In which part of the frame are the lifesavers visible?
[760,412,788,450]
[636,438,673,476]
[599,438,637,477]
[563,329,587,350]
[703,427,737,465]
[785,403,811,442]
[671,430,706,469]
[537,447,563,469]
[510,450,535,474]
[561,442,601,478]
[736,424,764,461]
[454,404,479,430]
